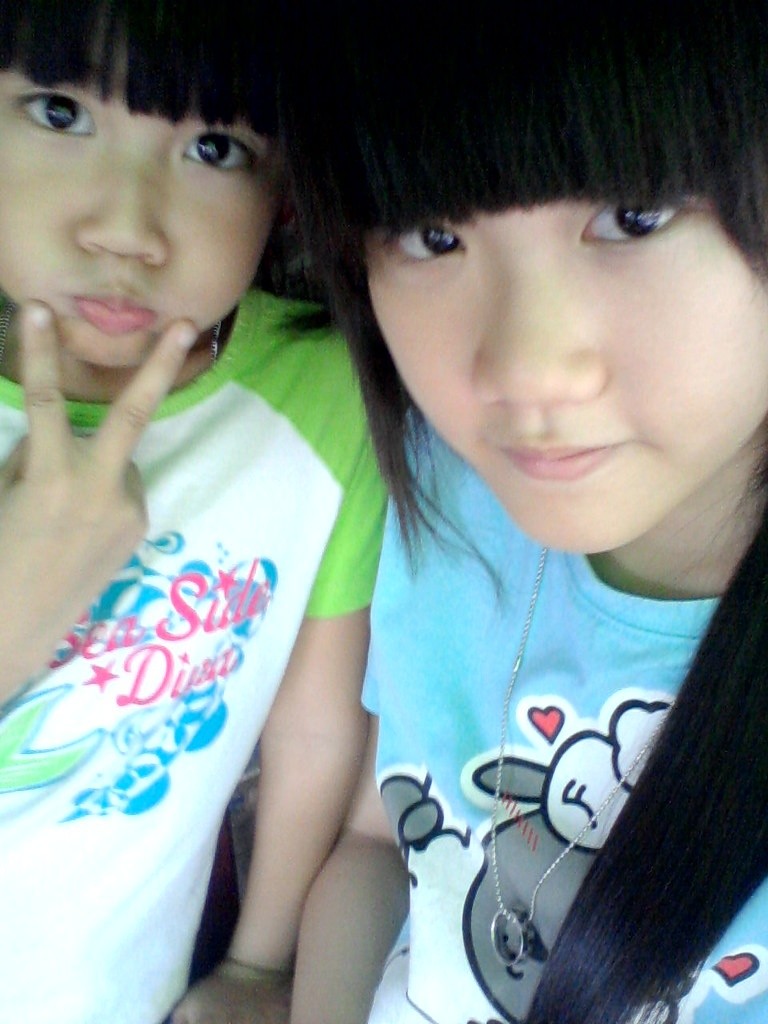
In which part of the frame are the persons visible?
[284,0,768,1024]
[0,0,381,1024]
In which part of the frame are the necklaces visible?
[486,548,682,971]
